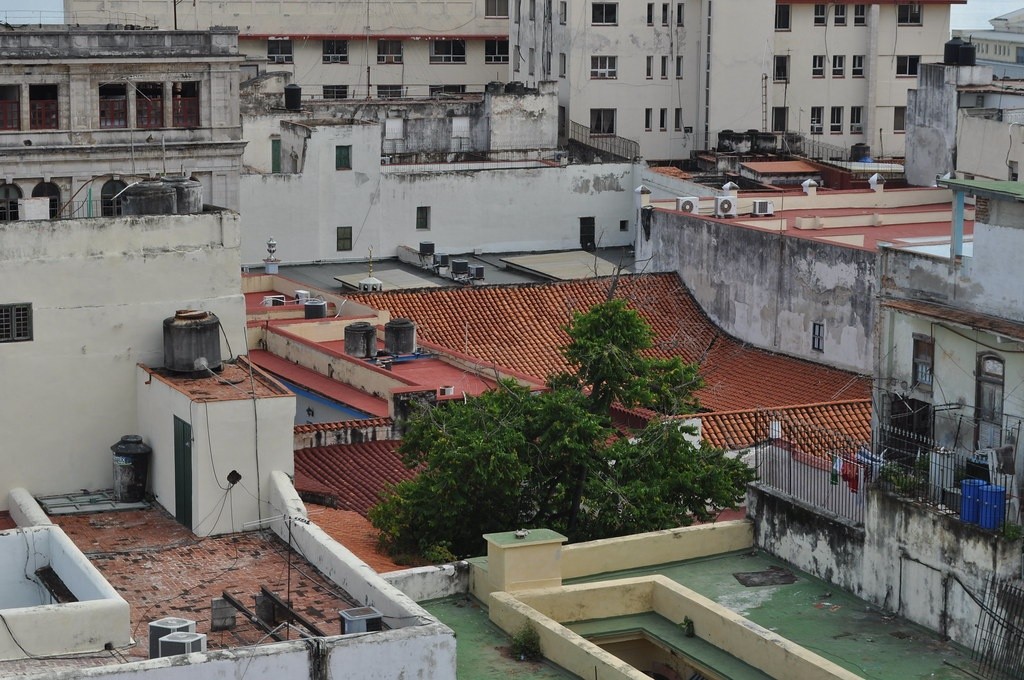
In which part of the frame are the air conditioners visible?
[381,157,391,165]
[753,200,774,214]
[714,196,737,215]
[263,295,285,307]
[295,289,310,304]
[676,196,699,215]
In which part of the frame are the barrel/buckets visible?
[978,485,1006,529]
[960,479,987,524]
